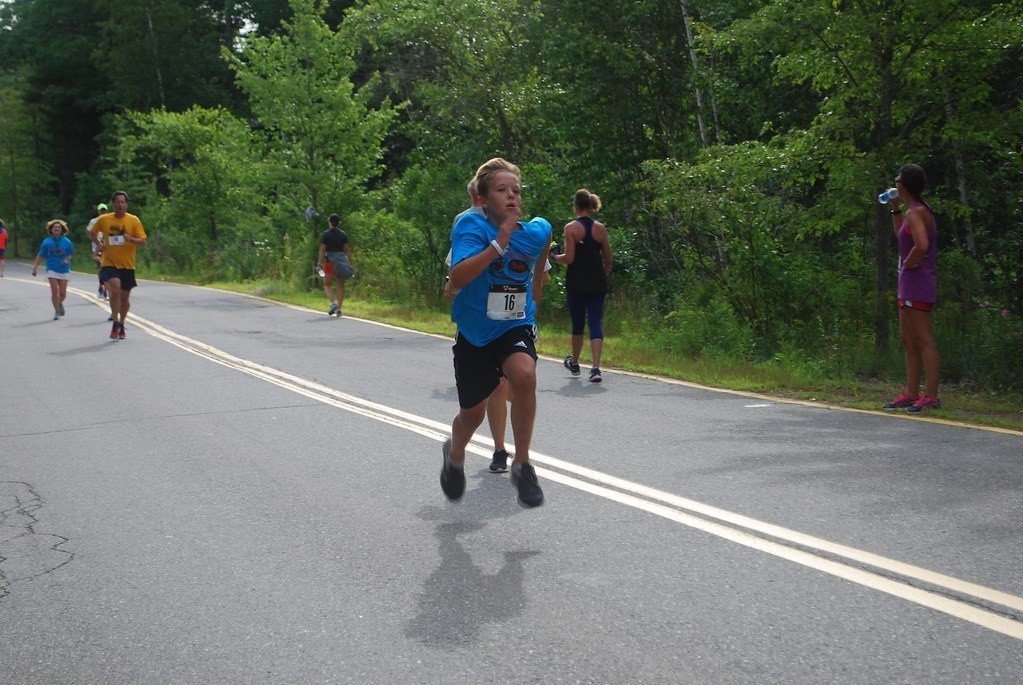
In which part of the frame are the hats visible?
[98,203,108,211]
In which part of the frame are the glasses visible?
[894,176,901,183]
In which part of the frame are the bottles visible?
[877,188,899,204]
[317,266,326,277]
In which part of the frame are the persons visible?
[89,190,147,339]
[551,188,612,382]
[446,177,551,472]
[317,213,352,317]
[882,163,940,413]
[441,158,572,508]
[32,219,75,320]
[86,203,110,300]
[0,219,7,276]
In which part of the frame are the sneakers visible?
[489,449,513,473]
[329,303,338,315]
[336,309,341,318]
[441,440,466,499]
[510,460,544,508]
[905,393,941,414]
[119,326,127,338]
[882,394,920,411]
[111,322,119,338]
[589,367,602,382]
[564,355,581,375]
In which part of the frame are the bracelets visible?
[131,237,134,242]
[66,257,69,260]
[317,263,321,266]
[890,209,902,214]
[491,240,505,256]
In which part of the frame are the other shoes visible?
[54,312,60,320]
[59,304,65,316]
[98,288,103,298]
[109,314,113,320]
[103,292,109,300]
